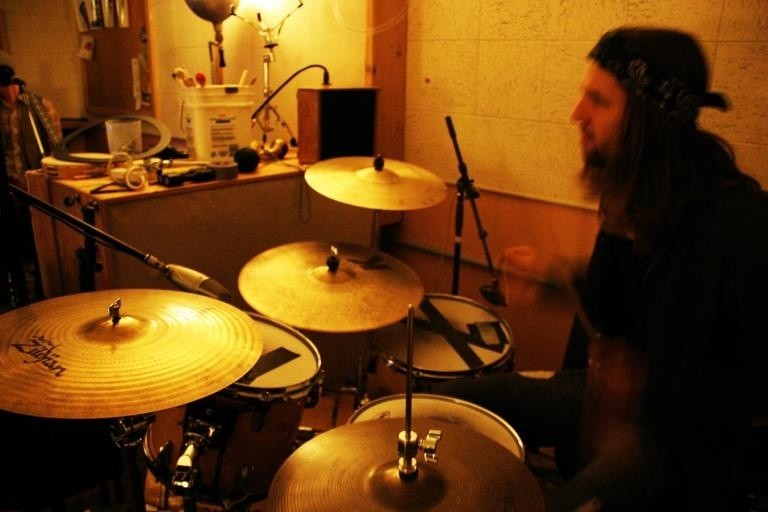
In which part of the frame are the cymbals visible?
[237,240,424,335]
[269,419,545,511]
[1,288,263,419]
[305,155,447,211]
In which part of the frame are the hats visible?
[591,31,724,117]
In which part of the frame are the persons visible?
[1,51,62,307]
[494,28,768,512]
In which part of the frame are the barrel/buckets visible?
[180,83,255,167]
[105,118,143,153]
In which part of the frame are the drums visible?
[359,292,517,402]
[347,394,526,463]
[144,311,324,500]
